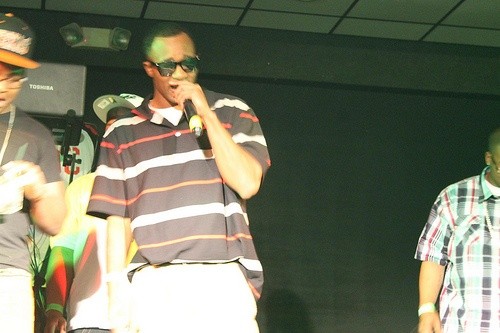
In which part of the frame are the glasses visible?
[490,157,500,174]
[149,56,198,77]
[0,70,31,87]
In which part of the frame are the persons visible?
[87,22,270,333]
[0,10,68,333]
[45,91,146,332]
[413,130,500,333]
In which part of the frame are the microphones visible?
[183,99,203,137]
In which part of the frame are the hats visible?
[0,12,42,70]
[92,92,144,124]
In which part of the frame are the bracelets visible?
[45,303,64,316]
[417,302,437,316]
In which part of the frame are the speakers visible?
[14,61,88,116]
